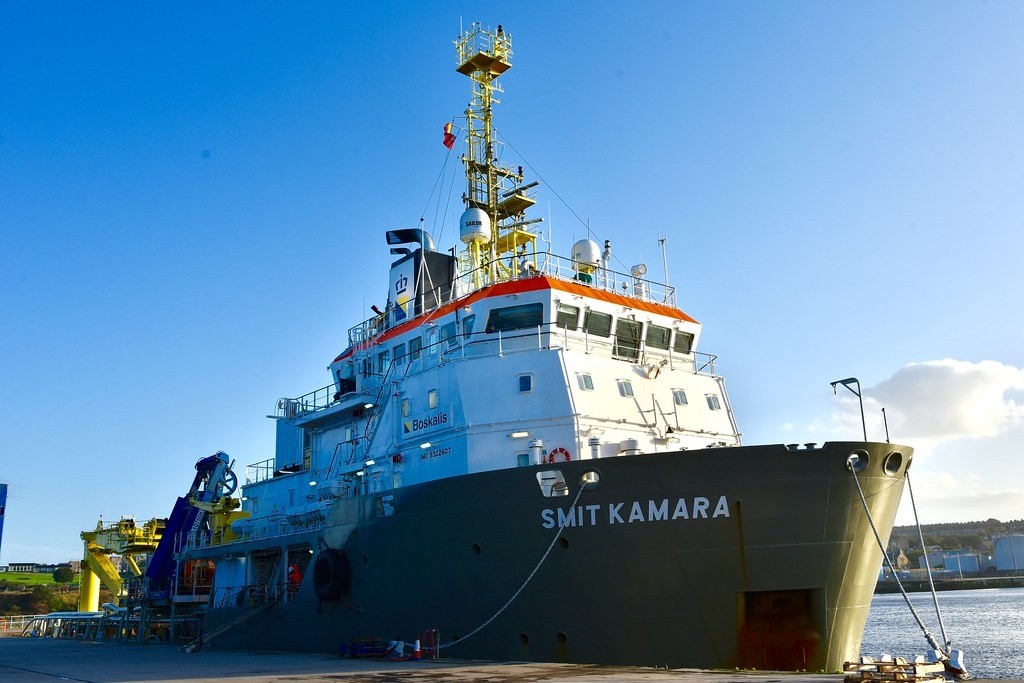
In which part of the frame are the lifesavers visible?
[549,448,571,465]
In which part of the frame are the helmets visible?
[288,566,293,573]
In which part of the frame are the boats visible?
[23,15,918,674]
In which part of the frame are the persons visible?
[59,584,68,592]
[286,565,303,595]
[150,623,170,640]
[186,561,215,592]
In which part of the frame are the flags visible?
[443,123,457,149]
